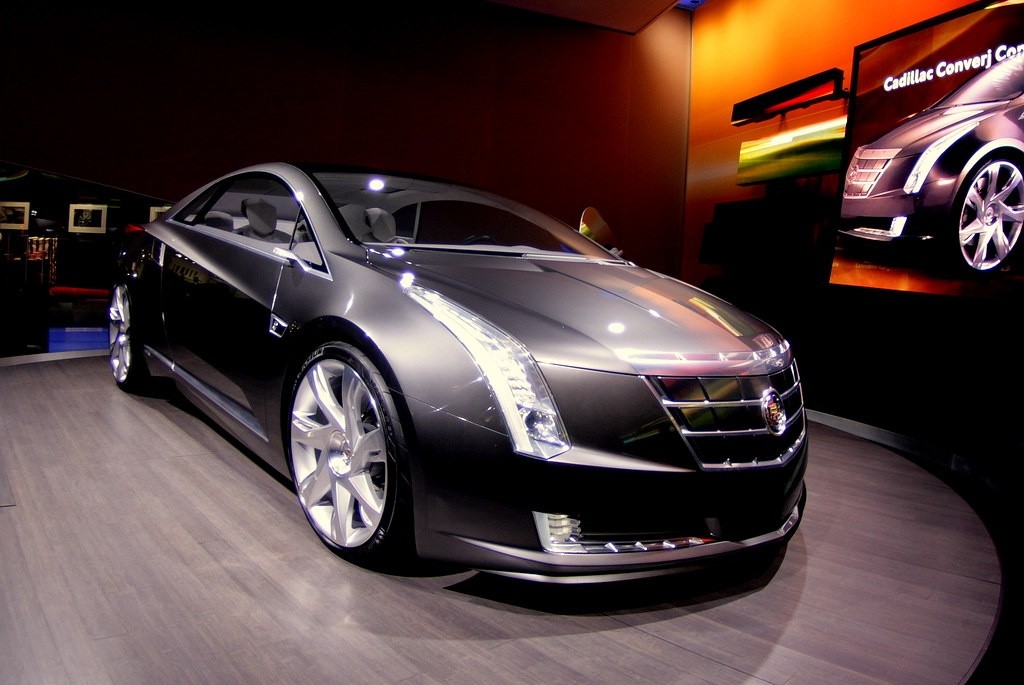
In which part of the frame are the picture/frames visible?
[68,204,107,234]
[0,202,30,230]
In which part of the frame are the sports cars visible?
[839,50,1024,283]
[108,162,811,586]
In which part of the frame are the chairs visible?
[231,198,298,243]
[360,208,409,244]
